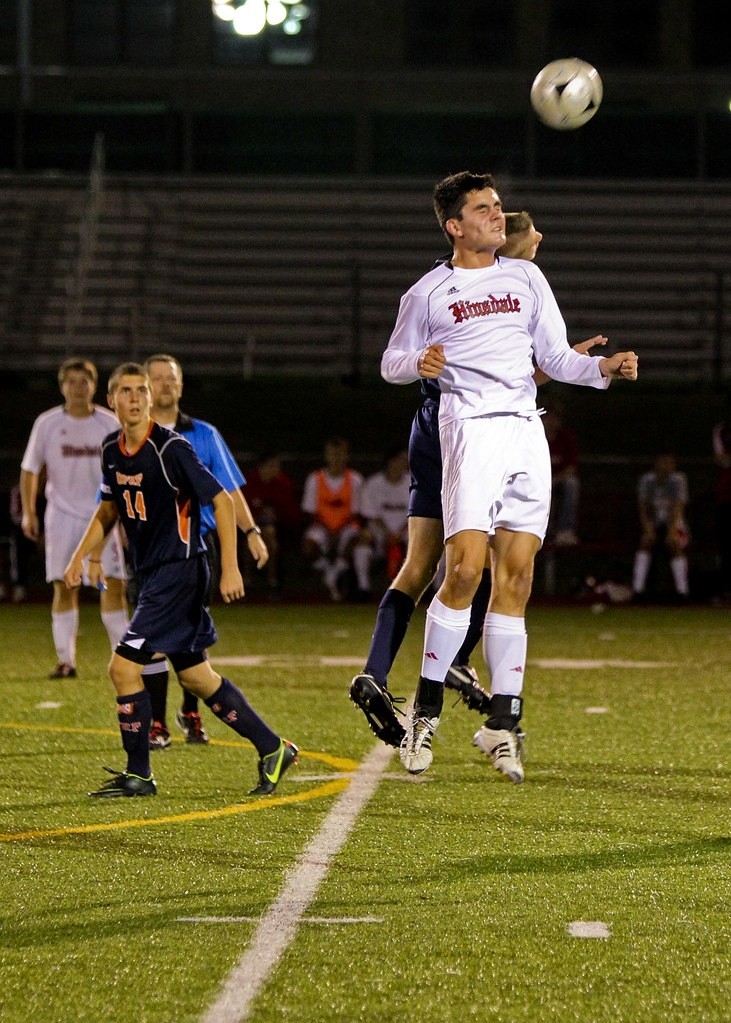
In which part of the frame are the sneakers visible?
[175,710,208,743]
[349,673,407,748]
[49,663,77,677]
[149,721,171,747]
[399,707,437,774]
[472,723,524,782]
[246,738,299,796]
[87,767,157,799]
[445,665,493,714]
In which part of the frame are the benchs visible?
[0,170,731,566]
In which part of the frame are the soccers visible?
[529,58,604,133]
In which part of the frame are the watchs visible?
[244,526,262,539]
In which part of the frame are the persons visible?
[379,171,639,784]
[350,212,607,750]
[3,440,730,609]
[85,353,269,749]
[62,363,299,799]
[19,355,131,680]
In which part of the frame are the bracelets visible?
[88,559,102,564]
